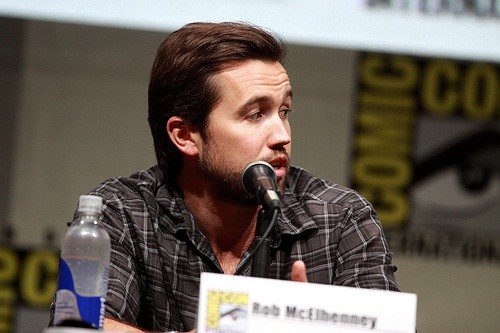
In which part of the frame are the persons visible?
[47,21,400,333]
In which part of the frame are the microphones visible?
[240,159,282,213]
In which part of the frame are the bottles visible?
[53,195,111,330]
